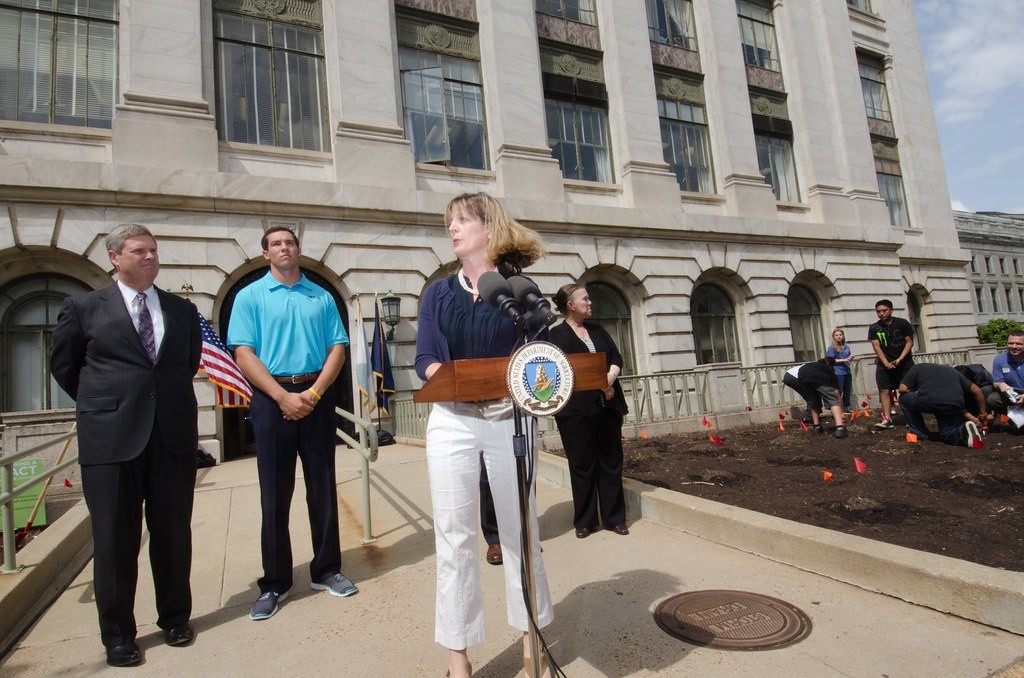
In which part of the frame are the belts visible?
[274,372,321,384]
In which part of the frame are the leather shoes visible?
[163,622,192,645]
[106,641,140,666]
[611,524,628,535]
[487,543,501,564]
[576,527,592,538]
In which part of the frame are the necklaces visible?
[458,267,499,294]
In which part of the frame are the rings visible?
[608,392,611,396]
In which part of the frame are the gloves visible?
[978,413,988,437]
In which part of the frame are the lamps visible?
[379,289,401,340]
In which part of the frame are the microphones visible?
[507,276,557,324]
[477,270,523,324]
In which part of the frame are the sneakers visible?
[250,587,293,620]
[875,413,894,428]
[311,572,357,596]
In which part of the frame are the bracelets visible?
[979,413,987,416]
[609,372,616,378]
[310,388,320,400]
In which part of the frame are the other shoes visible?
[814,425,823,432]
[834,426,849,437]
[963,420,985,449]
[803,416,814,424]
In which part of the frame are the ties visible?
[136,293,156,364]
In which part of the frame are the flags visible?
[370,300,402,415]
[356,300,377,414]
[197,312,253,408]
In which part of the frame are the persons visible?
[414,192,554,678]
[548,284,630,538]
[479,450,544,564]
[868,299,916,429]
[784,356,836,423]
[51,224,203,664]
[798,359,850,437]
[986,330,1024,429]
[826,328,855,414]
[953,366,994,435]
[898,363,987,448]
[227,226,359,620]
